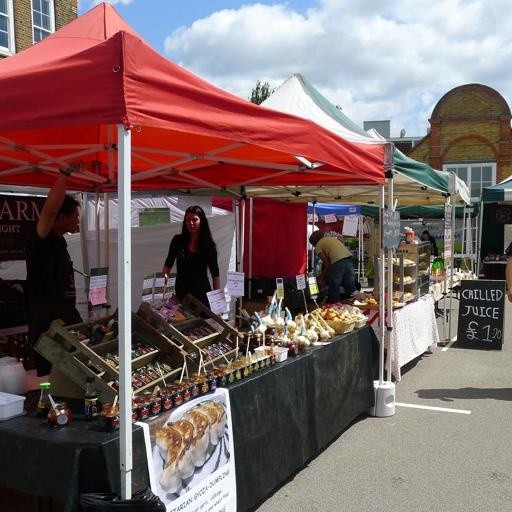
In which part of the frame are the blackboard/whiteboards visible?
[457,280,505,350]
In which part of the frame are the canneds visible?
[46,317,299,433]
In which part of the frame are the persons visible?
[421,231,438,257]
[163,206,220,309]
[309,231,357,303]
[505,240,512,302]
[25,161,85,377]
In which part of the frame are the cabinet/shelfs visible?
[377,244,418,302]
[417,242,432,298]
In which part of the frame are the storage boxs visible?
[0,392,26,418]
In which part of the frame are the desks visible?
[0,327,380,512]
[430,270,474,322]
[357,293,440,382]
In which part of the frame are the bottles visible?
[85,375,98,422]
[36,382,52,418]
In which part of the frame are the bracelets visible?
[58,168,70,177]
